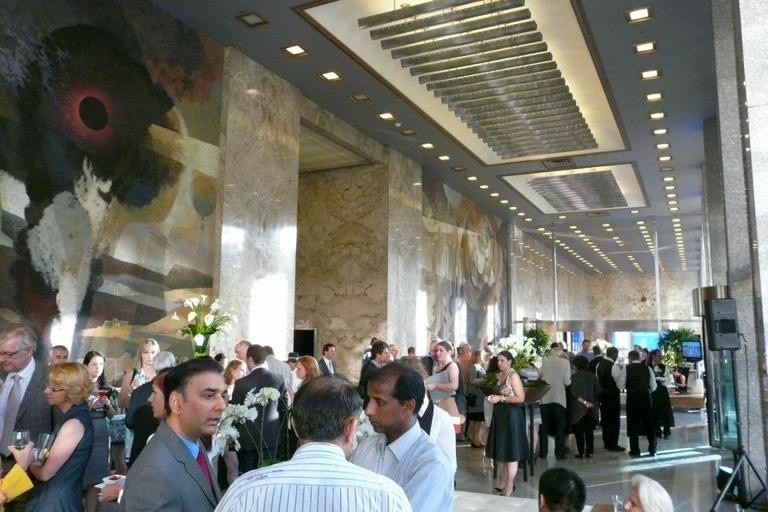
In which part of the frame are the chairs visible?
[675,367,690,393]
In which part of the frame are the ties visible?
[197,447,212,485]
[0,375,21,457]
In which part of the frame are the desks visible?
[492,402,542,483]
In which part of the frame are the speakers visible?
[702,296,741,352]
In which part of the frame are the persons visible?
[457,339,688,461]
[538,467,674,511]
[485,351,532,496]
[0,322,459,512]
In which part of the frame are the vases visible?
[470,377,550,402]
[190,339,208,360]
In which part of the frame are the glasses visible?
[48,384,64,392]
[0,350,19,357]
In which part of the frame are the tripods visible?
[708,359,768,512]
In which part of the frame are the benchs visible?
[668,395,705,412]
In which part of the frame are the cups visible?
[31,431,55,466]
[8,430,31,450]
[96,384,109,412]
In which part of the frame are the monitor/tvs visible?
[680,339,702,362]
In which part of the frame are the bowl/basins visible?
[102,473,126,487]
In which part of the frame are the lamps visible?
[716,464,736,495]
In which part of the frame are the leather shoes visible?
[585,453,593,458]
[650,451,656,456]
[609,446,625,451]
[575,454,584,458]
[495,485,515,493]
[470,442,486,448]
[629,450,640,455]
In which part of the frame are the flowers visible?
[216,387,302,465]
[172,294,238,355]
[487,335,538,380]
